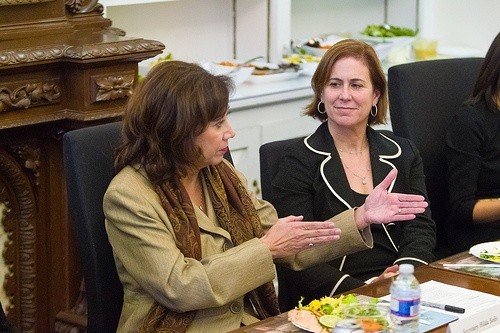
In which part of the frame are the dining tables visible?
[226,241,500,333]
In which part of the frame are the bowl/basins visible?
[195,63,255,86]
[343,303,388,333]
[297,36,416,63]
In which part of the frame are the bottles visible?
[389,264,421,333]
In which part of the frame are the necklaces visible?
[337,147,371,184]
[195,178,205,210]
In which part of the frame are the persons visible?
[102,60,428,333]
[267,38,438,314]
[426,32,500,262]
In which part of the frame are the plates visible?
[469,241,500,264]
[247,49,327,81]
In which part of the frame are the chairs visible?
[388,56,486,199]
[259,130,392,315]
[62,121,235,333]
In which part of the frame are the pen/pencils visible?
[420,301,465,313]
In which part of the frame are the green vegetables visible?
[300,48,307,55]
[479,250,500,259]
[295,294,389,328]
[361,23,419,37]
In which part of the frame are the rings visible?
[309,238,314,247]
[398,208,402,214]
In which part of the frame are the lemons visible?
[318,316,341,328]
